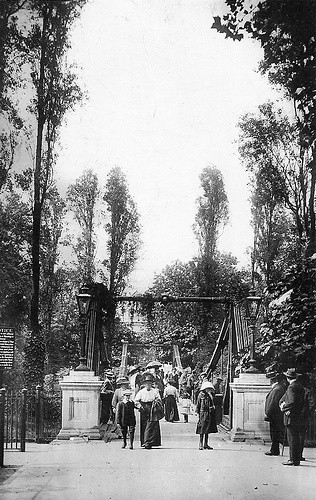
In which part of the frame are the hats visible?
[283,368,303,377]
[116,378,130,385]
[200,382,214,391]
[266,372,280,379]
[105,372,115,378]
[122,389,133,394]
[145,374,154,381]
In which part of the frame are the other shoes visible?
[122,444,126,448]
[265,452,280,456]
[204,444,213,450]
[129,447,133,449]
[145,445,151,449]
[199,444,203,450]
[282,461,299,465]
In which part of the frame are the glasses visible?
[126,395,130,396]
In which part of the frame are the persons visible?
[265,368,316,466]
[100,368,224,450]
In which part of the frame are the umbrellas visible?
[127,365,141,375]
[145,361,162,368]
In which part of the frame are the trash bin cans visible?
[213,394,223,426]
